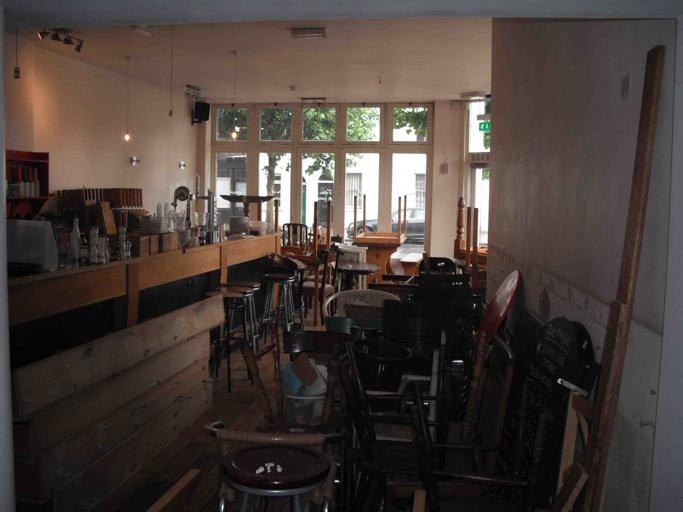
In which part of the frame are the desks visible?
[239,192,424,327]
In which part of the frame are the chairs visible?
[202,288,514,512]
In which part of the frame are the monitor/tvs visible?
[193,102,210,120]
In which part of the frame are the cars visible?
[346,208,425,243]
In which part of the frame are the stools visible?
[209,253,306,393]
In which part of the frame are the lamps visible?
[192,100,211,126]
[37,28,74,46]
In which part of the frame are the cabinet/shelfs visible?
[6,149,50,219]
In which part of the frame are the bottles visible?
[56,225,66,268]
[70,217,81,269]
[199,229,206,244]
[157,202,170,217]
[213,227,218,242]
